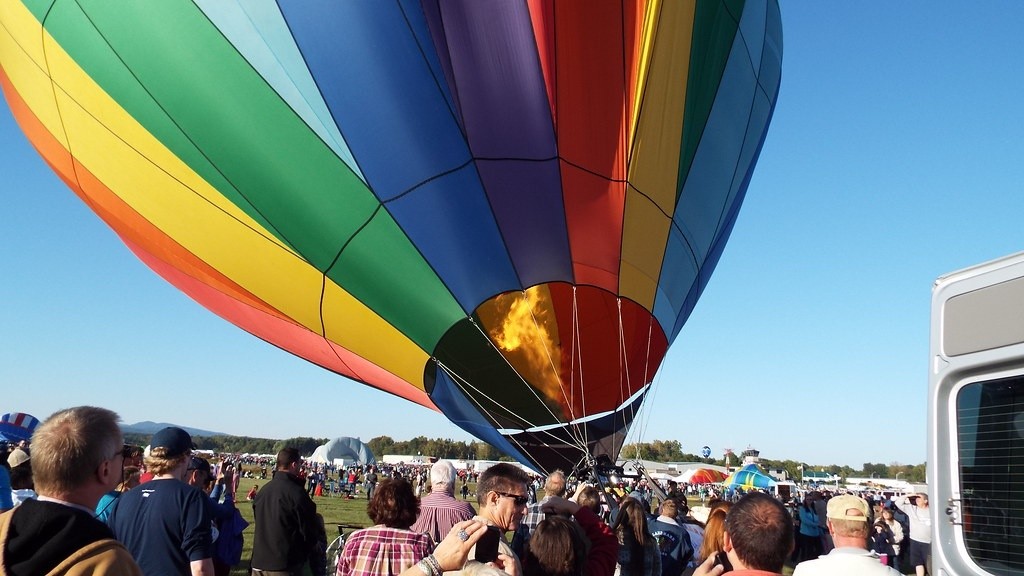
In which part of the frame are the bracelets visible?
[416,554,443,576]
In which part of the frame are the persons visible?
[0,405,933,576]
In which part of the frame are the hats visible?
[188,457,211,473]
[149,427,198,458]
[826,494,873,521]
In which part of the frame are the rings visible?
[458,530,468,541]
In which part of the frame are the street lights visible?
[895,472,903,487]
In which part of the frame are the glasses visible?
[115,445,132,458]
[495,492,529,506]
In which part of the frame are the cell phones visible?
[475,525,500,564]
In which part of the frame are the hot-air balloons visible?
[688,464,779,490]
[310,435,380,472]
[0,0,783,509]
